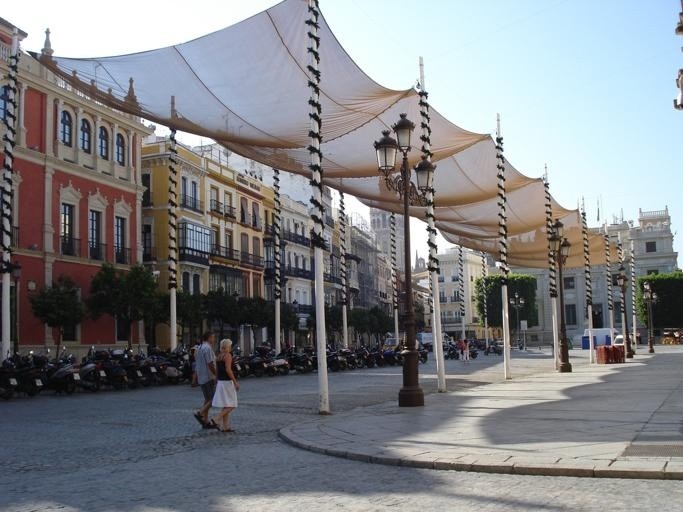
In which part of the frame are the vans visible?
[614,334,636,354]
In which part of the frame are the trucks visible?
[416,332,452,344]
[584,328,620,346]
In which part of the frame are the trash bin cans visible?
[605,335,611,345]
[582,336,597,349]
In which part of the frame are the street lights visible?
[616,263,628,358]
[547,218,572,372]
[373,113,435,407]
[510,292,526,349]
[643,281,657,353]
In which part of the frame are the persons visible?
[188,337,200,387]
[193,331,217,429]
[457,339,464,360]
[209,338,240,432]
[462,340,470,361]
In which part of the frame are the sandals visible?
[192,410,209,428]
[219,428,236,433]
[206,420,221,432]
